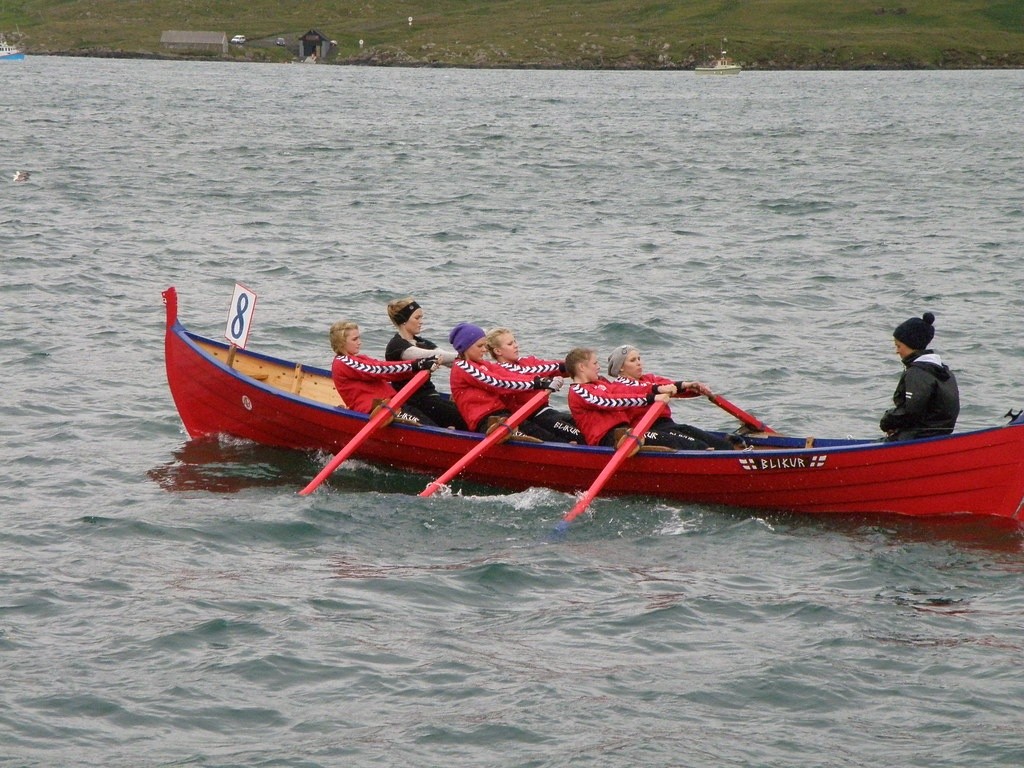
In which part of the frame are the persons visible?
[450,322,584,446]
[876,312,960,442]
[565,344,747,452]
[330,298,468,431]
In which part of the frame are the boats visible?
[163,286,1023,535]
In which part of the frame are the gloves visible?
[412,355,444,372]
[534,381,562,392]
[534,375,564,386]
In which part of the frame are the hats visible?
[449,323,485,353]
[892,312,935,350]
[607,345,636,377]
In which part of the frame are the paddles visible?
[693,382,779,436]
[299,355,444,497]
[549,387,672,543]
[418,373,564,500]
